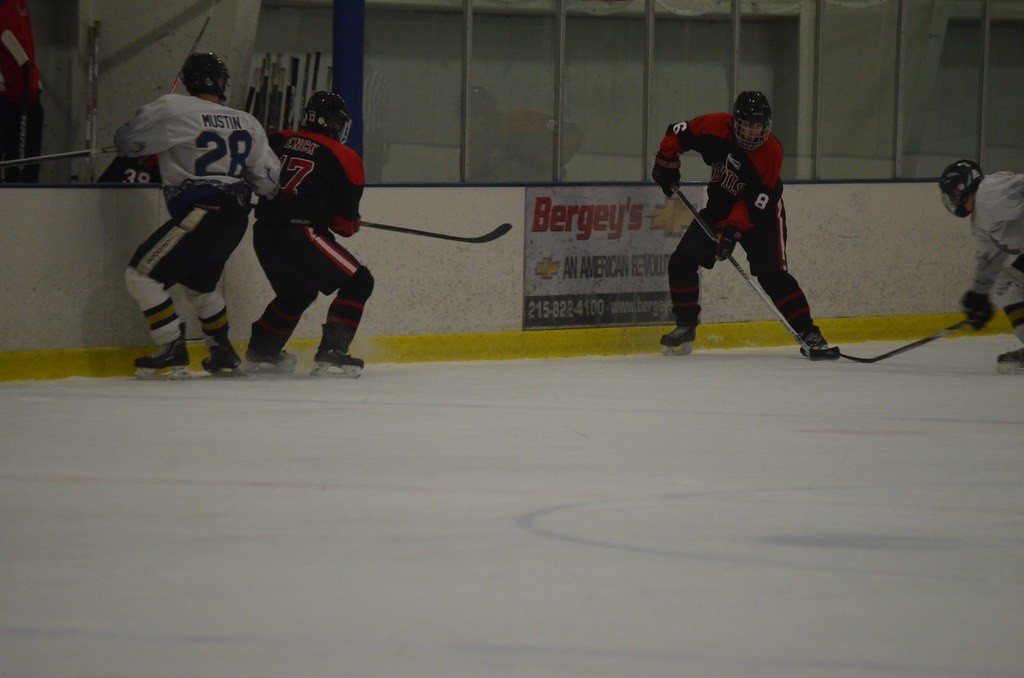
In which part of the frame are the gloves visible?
[960,291,997,330]
[714,225,742,261]
[651,152,682,199]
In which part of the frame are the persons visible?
[652,91,832,356]
[940,160,1024,375]
[244,90,374,379]
[114,53,282,379]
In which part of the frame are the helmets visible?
[939,159,984,218]
[733,89,773,151]
[180,52,232,105]
[304,91,352,144]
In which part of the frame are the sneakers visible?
[310,347,365,380]
[659,326,695,357]
[200,342,245,377]
[243,347,297,374]
[133,322,191,381]
[996,345,1023,377]
[799,325,828,356]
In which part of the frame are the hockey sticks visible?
[0,146,118,168]
[358,218,515,244]
[840,319,969,364]
[243,50,332,133]
[670,185,840,360]
[1,29,33,183]
[169,14,212,95]
[83,18,102,183]
